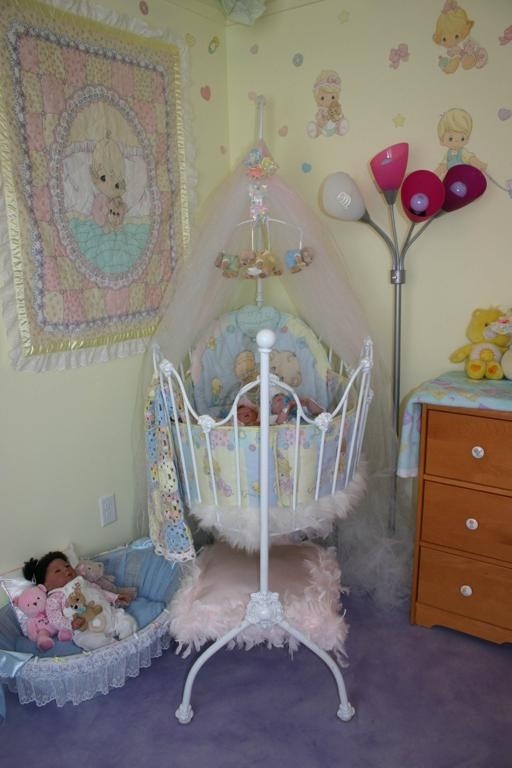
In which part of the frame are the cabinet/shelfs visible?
[411,404,512,649]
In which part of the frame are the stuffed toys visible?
[10,583,77,651]
[210,241,317,281]
[76,559,137,603]
[502,315,512,379]
[66,585,104,633]
[447,303,510,379]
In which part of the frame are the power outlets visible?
[99,494,118,528]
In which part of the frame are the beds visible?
[151,303,374,539]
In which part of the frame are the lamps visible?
[315,138,489,535]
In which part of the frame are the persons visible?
[22,550,141,654]
[268,385,325,426]
[230,397,264,427]
